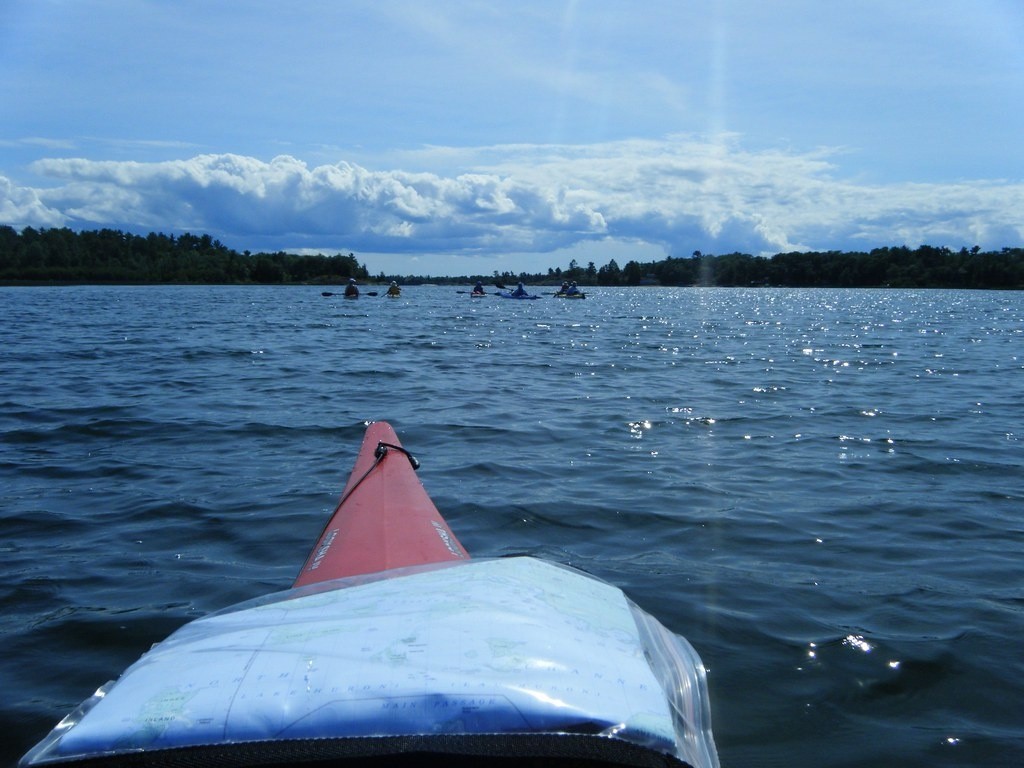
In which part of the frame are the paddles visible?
[380,274,417,298]
[541,291,590,295]
[496,282,523,294]
[457,290,507,297]
[320,289,380,297]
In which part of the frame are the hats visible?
[563,281,569,285]
[571,281,577,284]
[349,278,356,283]
[390,280,398,286]
[477,281,482,285]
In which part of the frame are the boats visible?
[500,292,536,299]
[557,291,585,298]
[387,293,400,298]
[471,293,488,297]
[344,293,358,299]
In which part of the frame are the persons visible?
[511,282,529,297]
[473,282,484,294]
[388,281,401,294]
[566,281,580,295]
[561,282,570,292]
[344,279,359,297]
